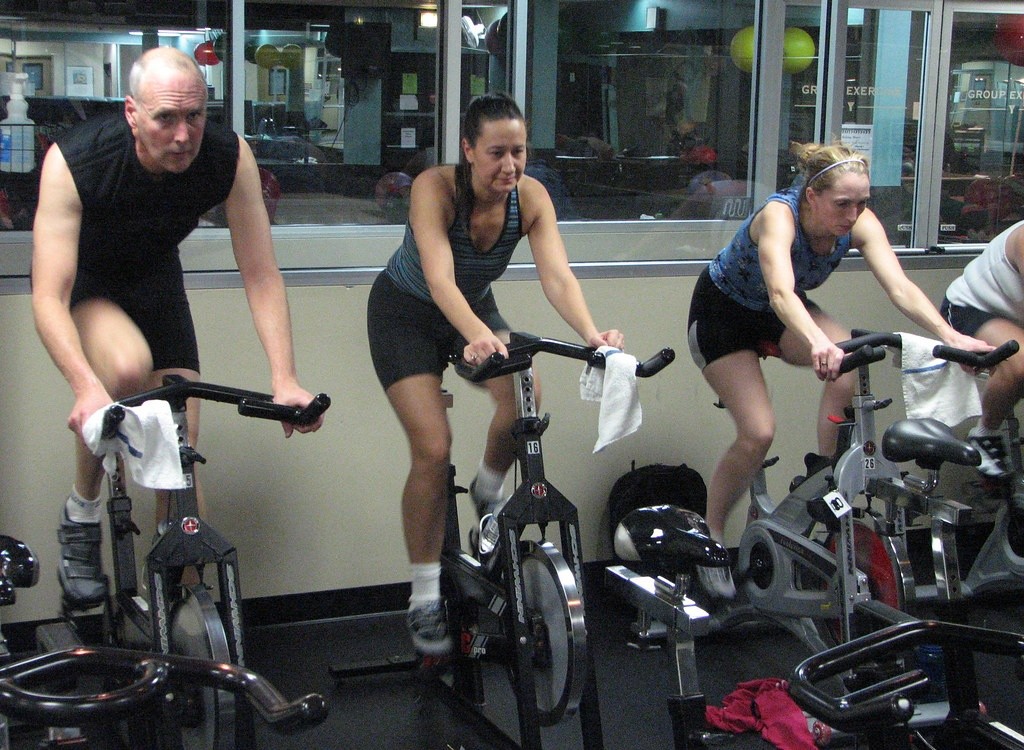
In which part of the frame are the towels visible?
[578,345,645,454]
[81,399,187,492]
[887,330,984,427]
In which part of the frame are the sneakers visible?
[965,427,1008,476]
[697,564,737,598]
[57,506,108,604]
[407,604,451,654]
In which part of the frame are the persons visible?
[940,219,1024,476]
[686,140,997,602]
[646,120,699,220]
[666,147,776,221]
[30,45,324,606]
[365,92,624,655]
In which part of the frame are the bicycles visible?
[0,534,329,750]
[33,375,332,750]
[738,325,1024,670]
[411,329,675,731]
[611,503,1024,733]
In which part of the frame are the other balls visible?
[781,28,815,74]
[731,25,753,72]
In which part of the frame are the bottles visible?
[482,494,514,551]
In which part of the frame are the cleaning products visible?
[0,67,39,176]
